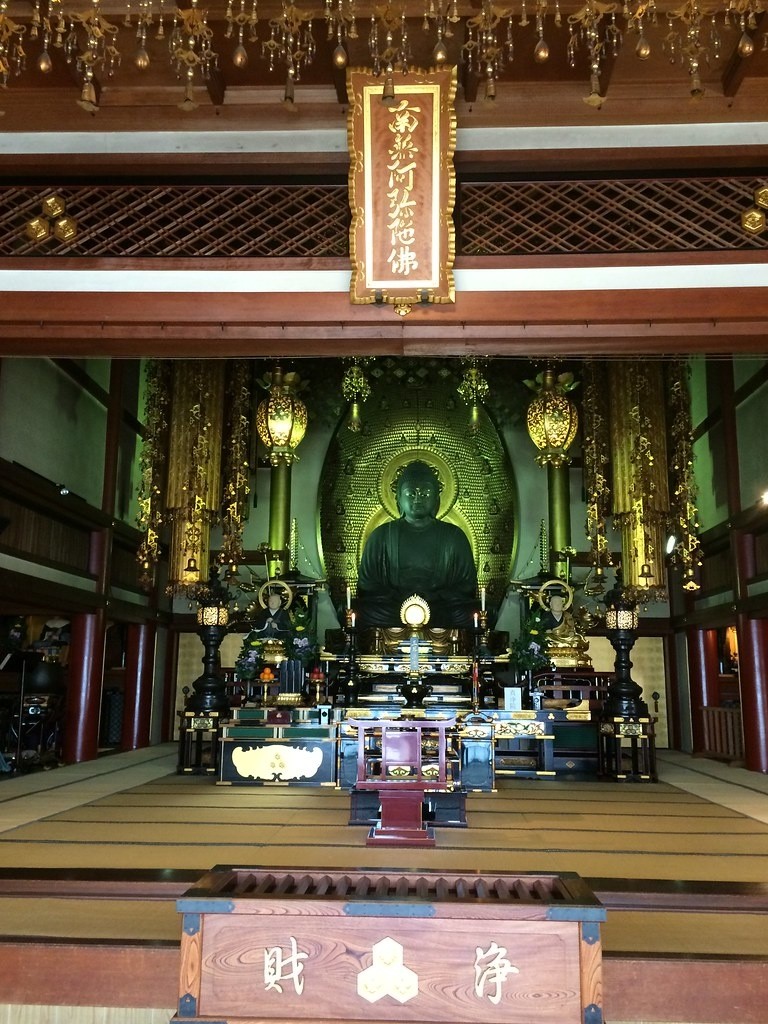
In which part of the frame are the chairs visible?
[346,715,458,846]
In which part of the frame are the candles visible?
[352,613,355,627]
[474,613,478,628]
[347,587,350,609]
[481,588,485,611]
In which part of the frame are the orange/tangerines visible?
[260,668,274,679]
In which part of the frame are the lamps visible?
[457,357,491,407]
[339,355,377,404]
[603,569,651,718]
[253,359,312,467]
[521,360,582,470]
[182,564,242,707]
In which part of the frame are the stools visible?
[175,710,232,776]
[599,716,659,784]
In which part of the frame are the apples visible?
[311,667,325,679]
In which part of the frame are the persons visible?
[356,459,479,627]
[256,594,292,639]
[542,596,576,636]
